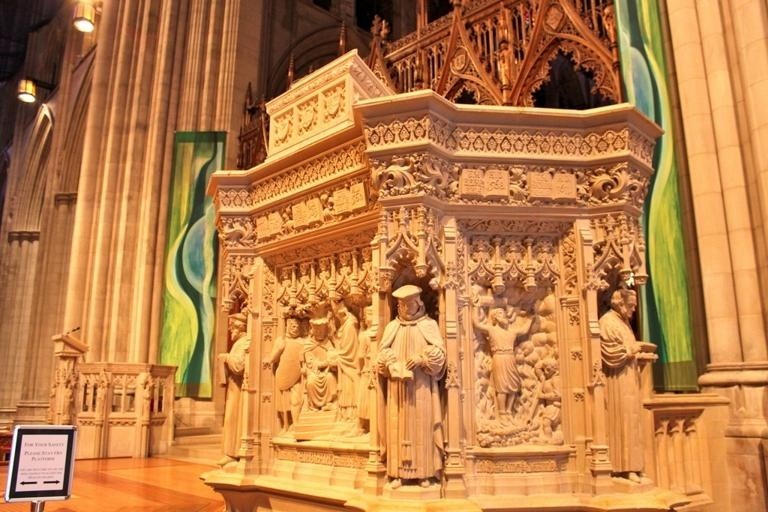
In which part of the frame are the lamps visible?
[16,75,57,104]
[72,0,102,33]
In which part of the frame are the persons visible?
[599,288,658,482]
[471,303,536,426]
[215,300,368,464]
[375,286,446,487]
